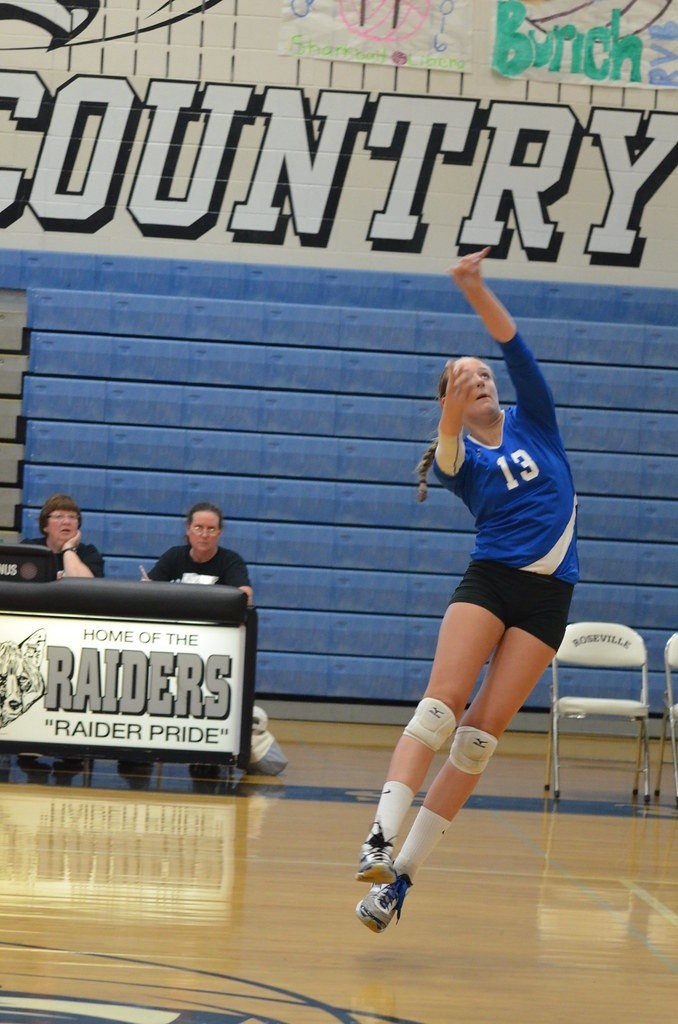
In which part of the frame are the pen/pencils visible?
[139,565,149,581]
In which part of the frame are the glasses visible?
[188,526,222,536]
[47,513,81,522]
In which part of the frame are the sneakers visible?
[355,862,413,932]
[356,822,397,885]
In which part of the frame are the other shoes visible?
[118,758,153,767]
[17,751,42,759]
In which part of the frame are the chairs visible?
[654,632,678,806]
[544,622,650,803]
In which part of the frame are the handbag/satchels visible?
[249,706,289,776]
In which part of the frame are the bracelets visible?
[61,547,77,556]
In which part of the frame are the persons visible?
[115,502,253,772]
[14,493,104,763]
[355,247,581,933]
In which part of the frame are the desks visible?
[0,576,259,790]
